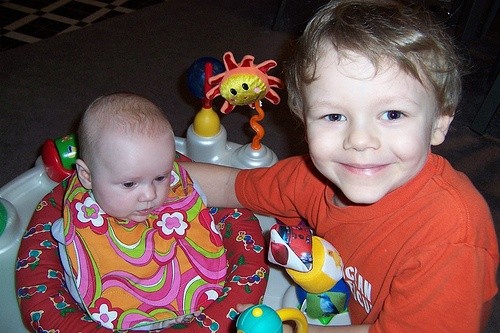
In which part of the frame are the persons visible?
[52,92,227,330]
[177,0,499,333]
